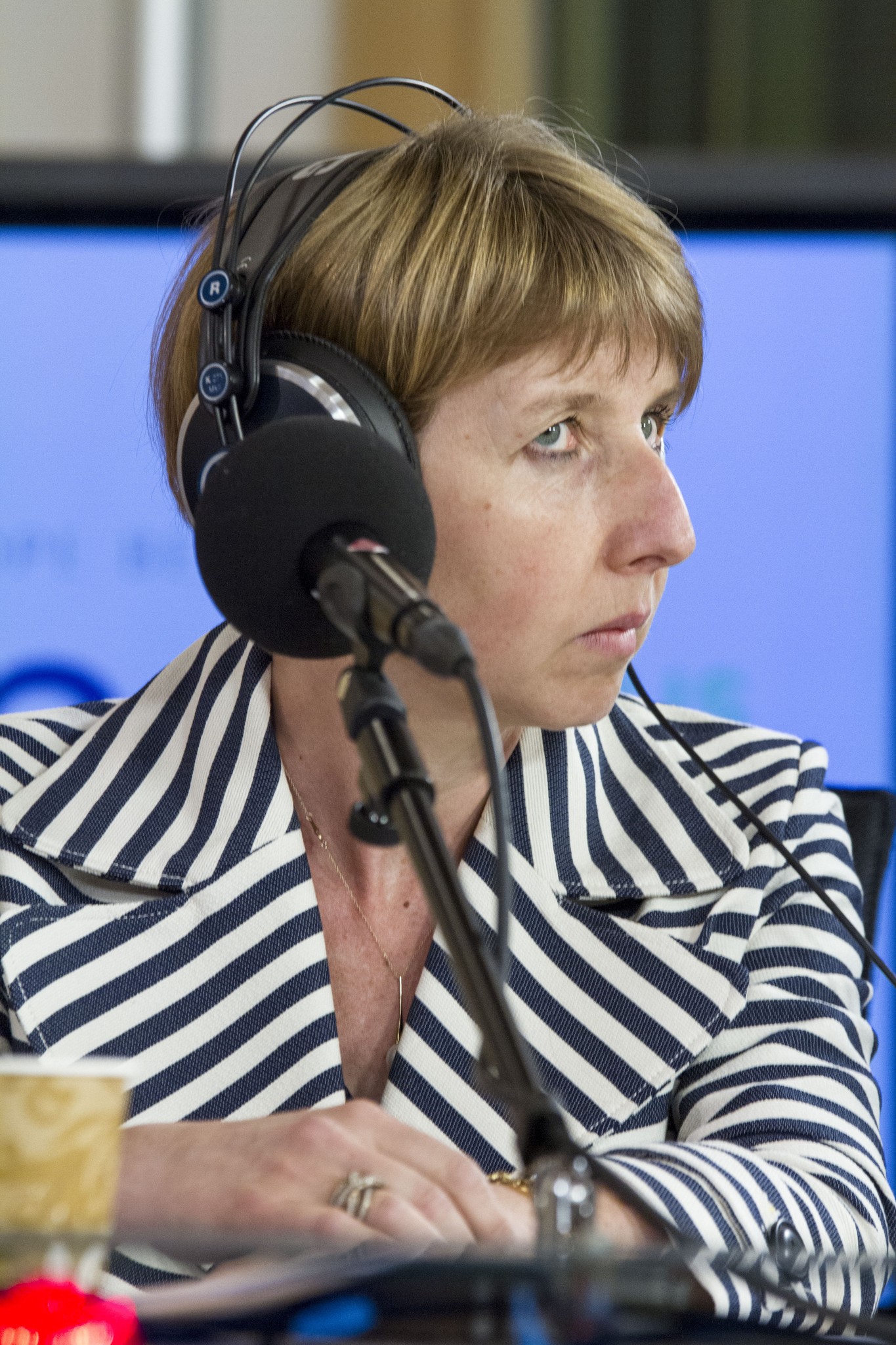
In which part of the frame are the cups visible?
[0,1055,127,1293]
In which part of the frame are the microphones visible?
[192,410,477,674]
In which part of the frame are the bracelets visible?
[478,1166,533,1199]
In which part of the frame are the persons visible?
[0,111,896,1345]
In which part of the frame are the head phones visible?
[174,81,485,524]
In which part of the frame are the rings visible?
[329,1171,388,1223]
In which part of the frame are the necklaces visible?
[272,758,436,1047]
